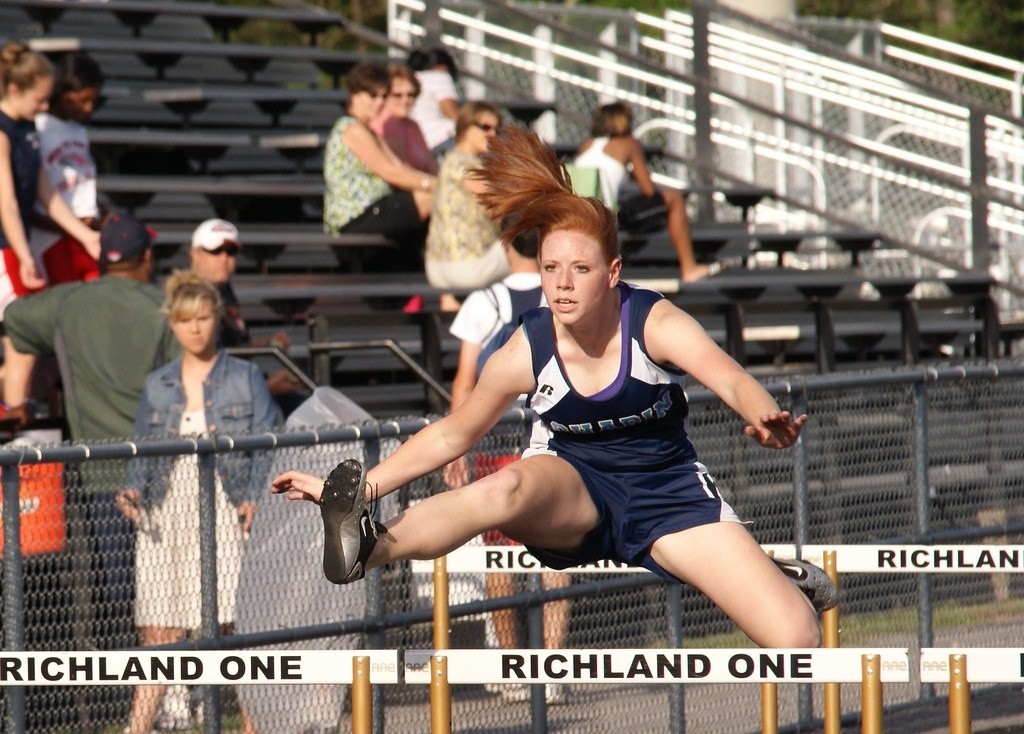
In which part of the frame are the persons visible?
[371,63,438,175]
[573,103,714,283]
[269,126,839,649]
[408,48,462,159]
[322,58,461,311]
[442,206,571,706]
[32,48,103,287]
[116,272,285,733]
[167,218,251,362]
[425,101,539,291]
[0,41,56,430]
[4,216,171,606]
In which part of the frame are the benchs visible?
[0,0,1024,410]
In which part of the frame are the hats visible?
[100,213,158,264]
[192,218,241,251]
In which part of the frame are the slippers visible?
[710,261,725,278]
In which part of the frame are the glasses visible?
[389,91,418,98]
[360,83,388,99]
[202,243,238,256]
[472,120,500,135]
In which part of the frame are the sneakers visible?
[319,458,388,585]
[769,555,839,615]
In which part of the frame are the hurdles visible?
[405,541,1024,734]
[0,632,1024,734]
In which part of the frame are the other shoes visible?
[544,681,571,705]
[502,683,532,704]
[154,684,204,731]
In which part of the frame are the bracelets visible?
[422,175,430,189]
